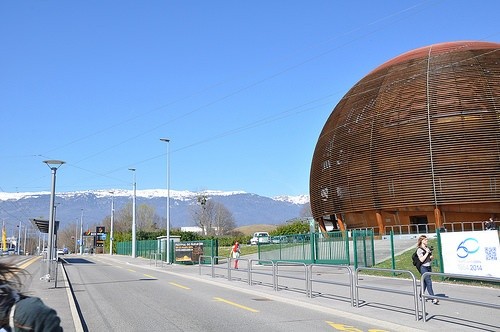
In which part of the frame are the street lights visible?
[159,138,171,265]
[128,168,136,259]
[42,159,66,283]
[75,217,78,254]
[109,192,114,255]
[80,208,84,256]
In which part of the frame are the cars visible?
[272,234,311,244]
[63,248,69,254]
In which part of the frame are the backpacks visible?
[412,246,426,266]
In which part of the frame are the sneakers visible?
[420,297,429,301]
[431,299,439,304]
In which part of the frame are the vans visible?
[249,231,271,245]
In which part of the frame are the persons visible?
[229,239,240,269]
[416,235,439,304]
[485,218,496,230]
[0,263,64,332]
